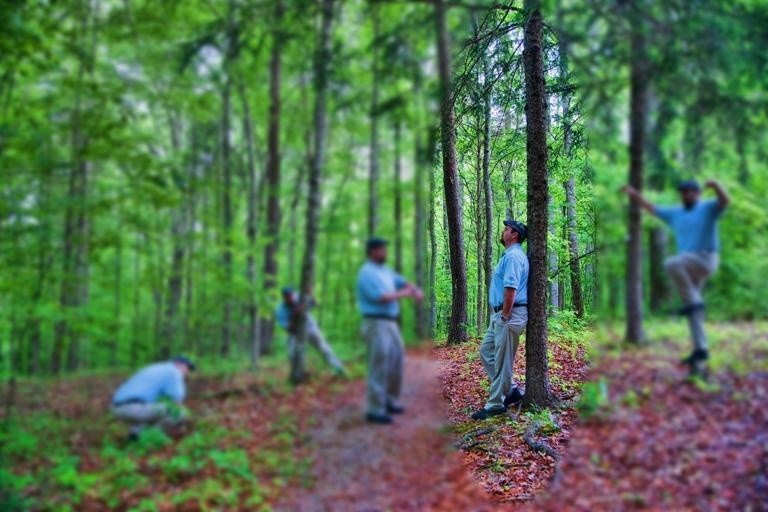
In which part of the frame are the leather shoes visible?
[503,387,522,406]
[386,405,403,415]
[681,349,708,365]
[364,412,394,425]
[472,405,506,420]
[678,303,703,315]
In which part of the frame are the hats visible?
[677,181,697,191]
[174,356,194,370]
[503,220,528,241]
[365,236,389,248]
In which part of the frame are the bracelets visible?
[412,287,419,296]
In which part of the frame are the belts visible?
[365,313,395,320]
[494,303,527,312]
[115,398,143,406]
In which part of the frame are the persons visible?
[613,175,731,379]
[272,284,351,381]
[350,237,426,425]
[105,355,198,449]
[469,219,529,419]
